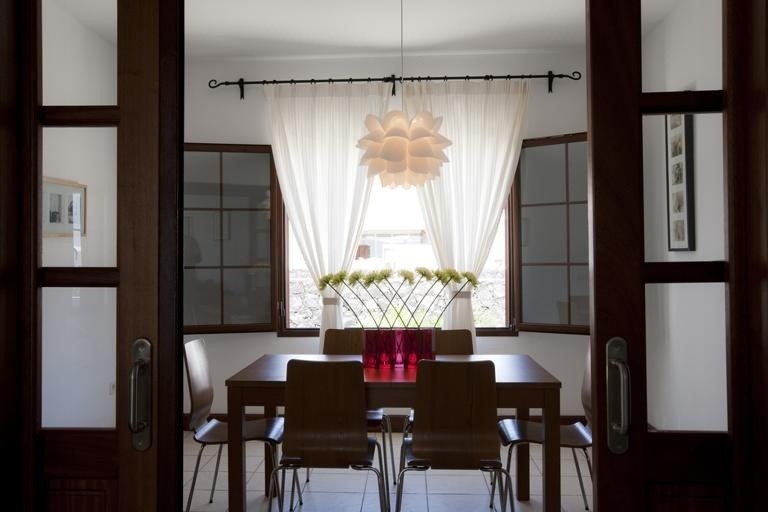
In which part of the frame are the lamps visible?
[354,1,456,192]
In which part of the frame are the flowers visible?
[318,266,481,328]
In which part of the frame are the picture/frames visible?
[665,112,696,252]
[41,176,88,239]
